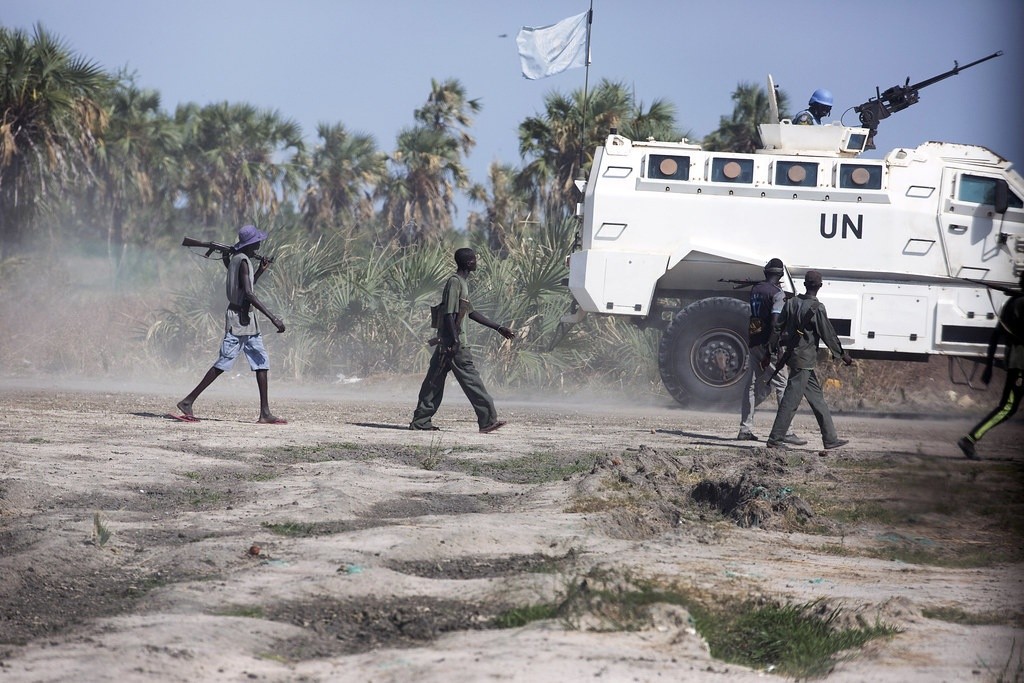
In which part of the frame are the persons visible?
[791,88,833,124]
[176,224,287,424]
[759,270,851,450]
[737,258,809,446]
[957,271,1024,461]
[408,247,516,434]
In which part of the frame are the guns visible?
[853,50,1005,138]
[766,306,815,387]
[717,277,785,290]
[428,298,471,390]
[180,237,275,268]
[961,275,1024,297]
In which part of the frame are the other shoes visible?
[766,441,793,451]
[479,419,506,434]
[784,433,808,445]
[823,439,849,449]
[738,432,759,441]
[957,438,981,460]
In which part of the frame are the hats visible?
[233,225,268,250]
[805,271,822,286]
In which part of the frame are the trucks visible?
[560,47,1024,414]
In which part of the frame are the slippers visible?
[257,420,287,425]
[170,412,200,422]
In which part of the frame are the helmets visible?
[809,89,833,109]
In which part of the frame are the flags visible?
[516,10,588,79]
